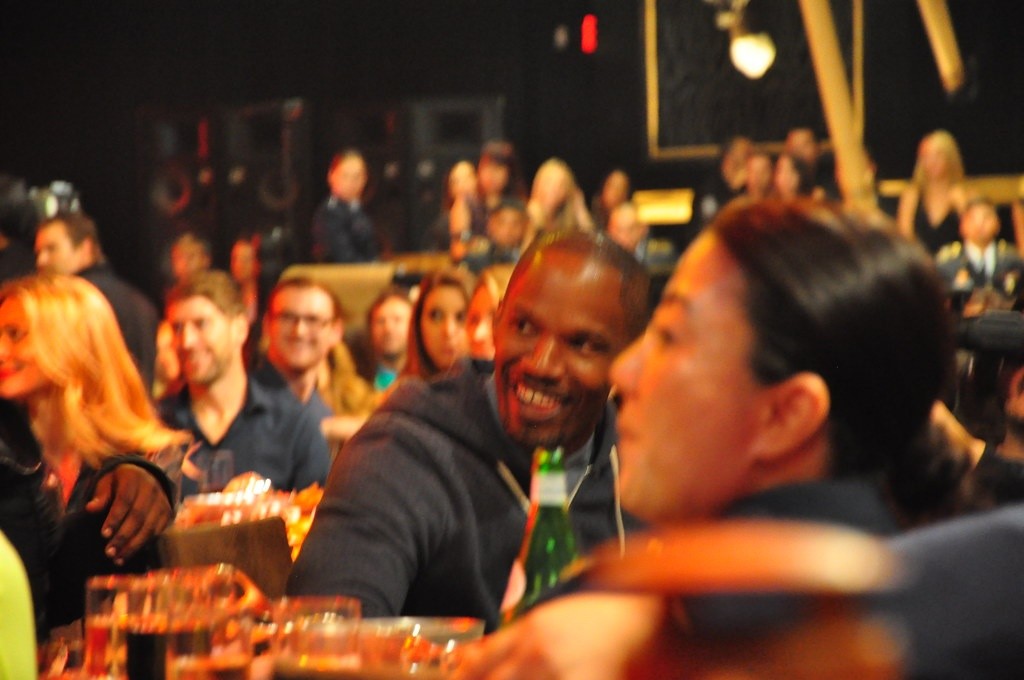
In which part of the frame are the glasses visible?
[271,310,321,324]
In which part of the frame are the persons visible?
[154,270,330,504]
[251,277,344,424]
[397,272,470,391]
[0,274,202,505]
[174,131,1023,511]
[287,230,650,637]
[456,201,956,680]
[465,264,515,361]
[0,398,179,673]
[354,292,414,392]
[35,213,160,398]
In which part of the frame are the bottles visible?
[501,442,584,627]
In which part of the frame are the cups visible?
[80,564,485,680]
